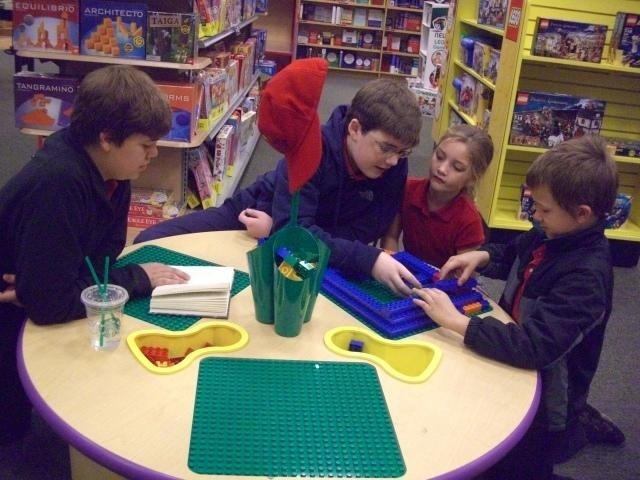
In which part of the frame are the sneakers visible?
[581,405,624,444]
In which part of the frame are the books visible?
[148,265,236,317]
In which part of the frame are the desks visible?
[14,230,543,479]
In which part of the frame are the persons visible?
[133,79,423,297]
[382,125,494,281]
[413,135,626,478]
[0,64,191,478]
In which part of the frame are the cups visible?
[80,284,130,349]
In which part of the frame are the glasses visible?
[366,132,413,158]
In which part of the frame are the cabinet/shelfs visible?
[428,1,640,267]
[291,0,426,80]
[12,0,279,228]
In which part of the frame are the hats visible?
[258,58,328,192]
